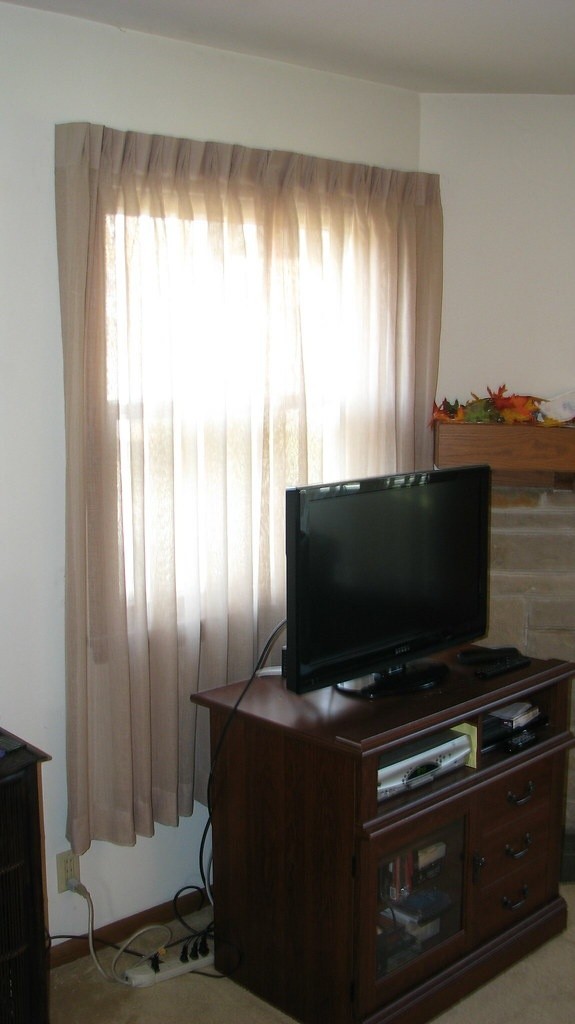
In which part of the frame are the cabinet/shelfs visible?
[0,727,55,1024]
[189,644,575,1024]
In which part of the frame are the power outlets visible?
[56,850,81,892]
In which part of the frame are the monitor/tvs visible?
[285,463,492,699]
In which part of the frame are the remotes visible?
[473,657,531,680]
[457,646,524,666]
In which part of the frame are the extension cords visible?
[125,951,215,987]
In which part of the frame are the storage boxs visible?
[491,471,557,488]
[434,421,575,473]
